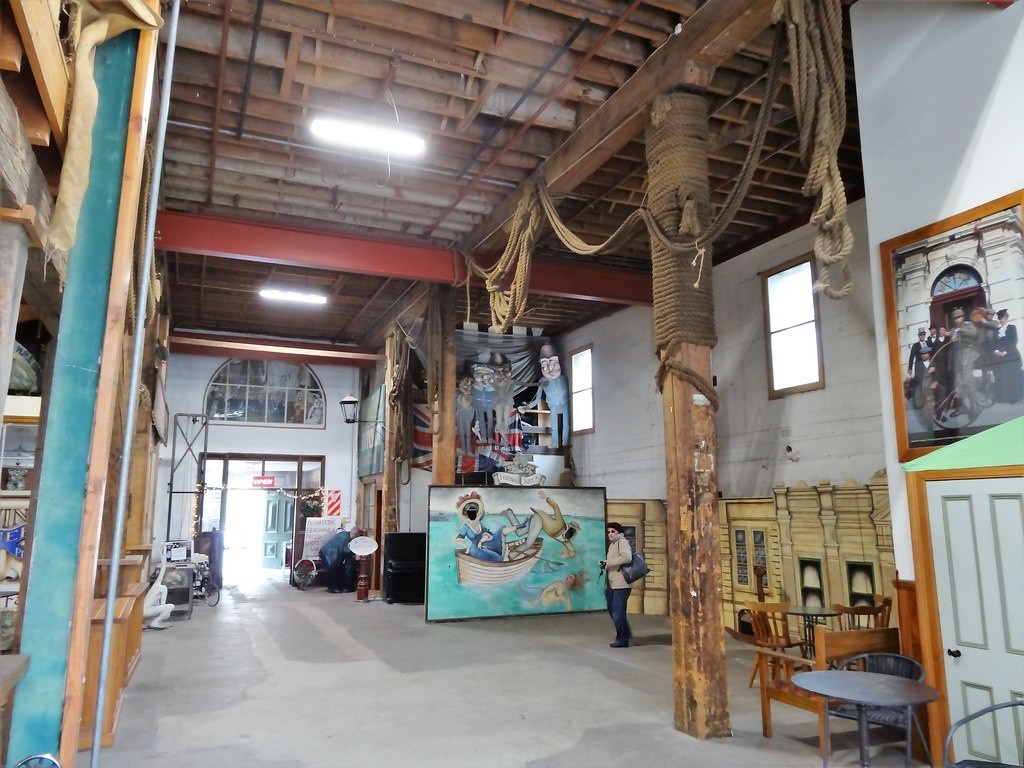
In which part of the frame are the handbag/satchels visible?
[618,538,650,582]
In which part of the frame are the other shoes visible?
[951,410,960,418]
[327,587,342,593]
[936,412,946,422]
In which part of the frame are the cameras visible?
[600,561,606,575]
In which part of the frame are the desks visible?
[785,605,843,671]
[790,670,940,768]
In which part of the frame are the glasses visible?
[607,530,618,534]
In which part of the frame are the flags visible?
[406,383,533,477]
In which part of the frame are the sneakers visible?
[616,630,632,640]
[610,639,629,647]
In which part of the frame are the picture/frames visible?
[880,189,1024,462]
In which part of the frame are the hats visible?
[917,327,927,335]
[928,324,937,330]
[919,348,929,354]
[987,309,997,315]
[952,308,965,319]
[997,308,1009,320]
[606,522,624,533]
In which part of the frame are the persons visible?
[317,525,364,595]
[597,520,636,652]
[903,299,1023,425]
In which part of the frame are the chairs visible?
[756,625,901,761]
[830,593,893,672]
[942,699,1024,768]
[823,652,936,768]
[743,599,813,688]
